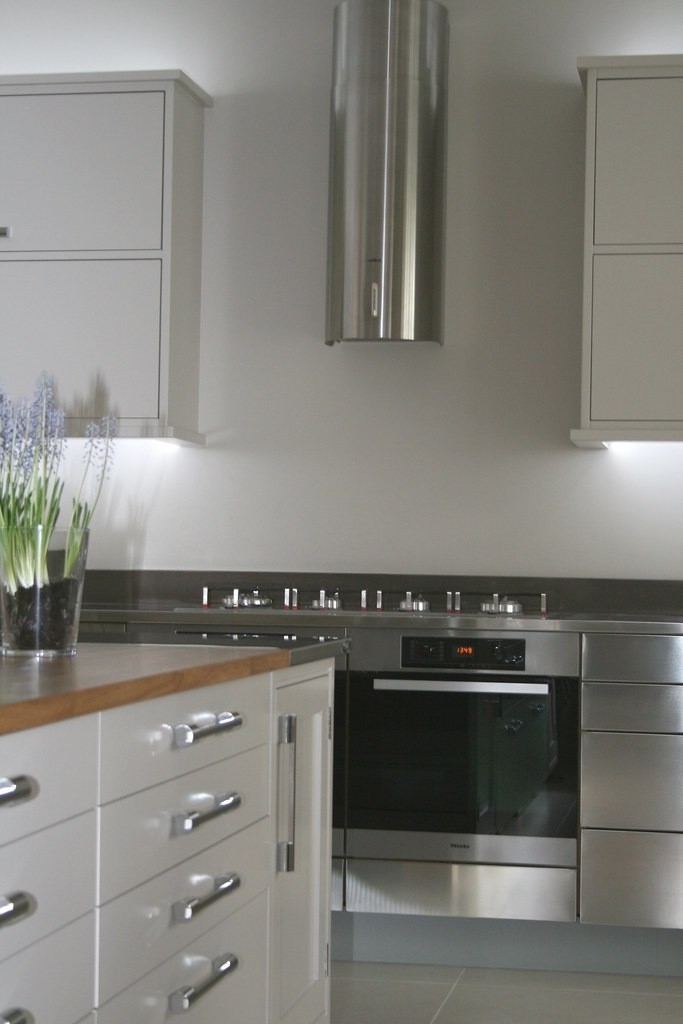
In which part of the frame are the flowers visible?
[0,378,122,584]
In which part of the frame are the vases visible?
[2,577,83,657]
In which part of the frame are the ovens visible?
[345,627,579,926]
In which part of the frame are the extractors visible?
[324,2,442,349]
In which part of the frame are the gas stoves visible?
[174,574,548,630]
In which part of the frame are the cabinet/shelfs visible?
[566,54,682,444]
[1,66,212,443]
[0,658,336,1022]
